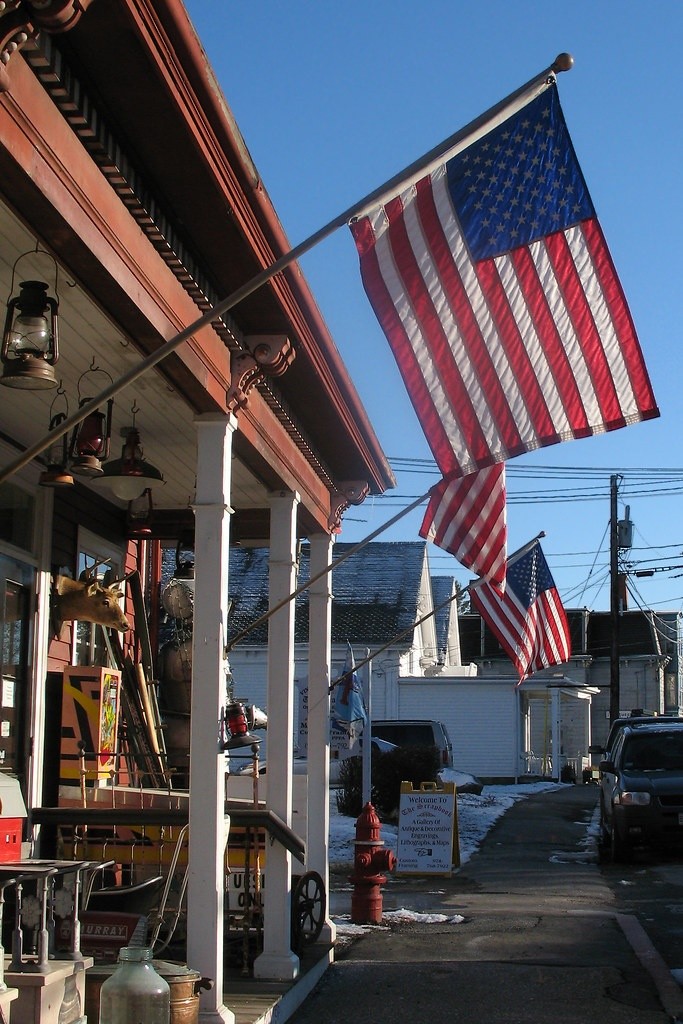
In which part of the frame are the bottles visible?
[99,947,171,1024]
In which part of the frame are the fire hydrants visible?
[347,801,396,925]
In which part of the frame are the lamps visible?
[70,353,119,476]
[0,222,66,392]
[38,374,75,488]
[90,393,167,500]
[173,500,194,581]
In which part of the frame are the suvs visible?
[360,720,455,774]
[587,715,683,860]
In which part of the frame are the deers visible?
[53,554,138,636]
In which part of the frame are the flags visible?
[424,461,514,595]
[467,537,572,682]
[343,67,650,479]
